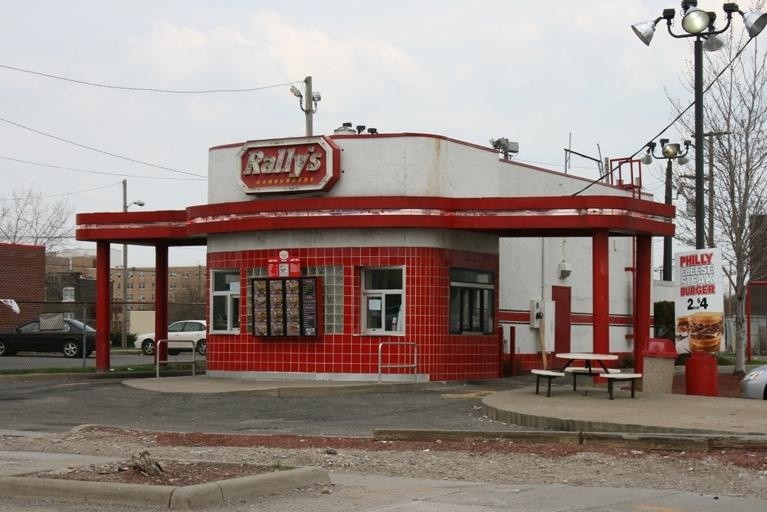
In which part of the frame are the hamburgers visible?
[689,312,723,347]
[677,316,689,332]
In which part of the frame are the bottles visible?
[391,317,396,330]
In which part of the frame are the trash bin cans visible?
[640,338,679,394]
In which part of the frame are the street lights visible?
[120,179,146,349]
[630,1,766,281]
[642,138,691,281]
[290,76,324,138]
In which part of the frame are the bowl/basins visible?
[641,337,679,358]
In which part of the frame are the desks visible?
[554,352,619,374]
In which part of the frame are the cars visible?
[0,317,98,358]
[133,319,208,355]
[739,365,766,399]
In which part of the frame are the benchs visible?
[531,367,642,400]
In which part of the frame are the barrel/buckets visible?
[686,351,720,397]
